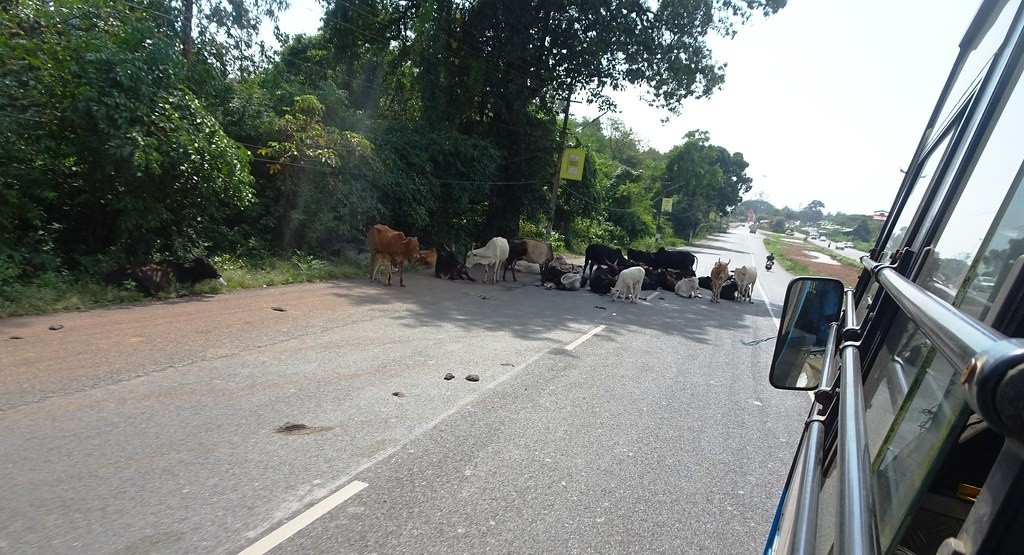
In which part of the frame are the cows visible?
[102,249,227,297]
[364,223,757,307]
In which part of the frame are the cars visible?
[819,235,826,242]
[973,275,996,292]
[845,241,854,248]
[835,243,845,250]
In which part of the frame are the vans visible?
[811,232,818,240]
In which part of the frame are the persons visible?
[766,253,775,265]
[828,242,831,247]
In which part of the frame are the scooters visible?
[765,261,772,270]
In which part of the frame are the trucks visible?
[769,0,1024,555]
